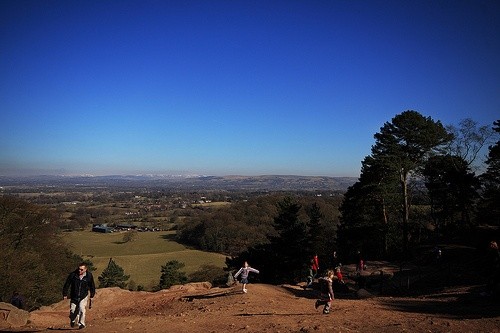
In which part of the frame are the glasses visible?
[78,267,84,271]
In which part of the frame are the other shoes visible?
[71,320,75,327]
[242,288,247,293]
[79,324,85,329]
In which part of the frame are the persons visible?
[336,268,342,283]
[356,257,365,271]
[63,263,96,329]
[307,254,320,287]
[9,290,23,309]
[315,269,334,315]
[234,261,260,294]
